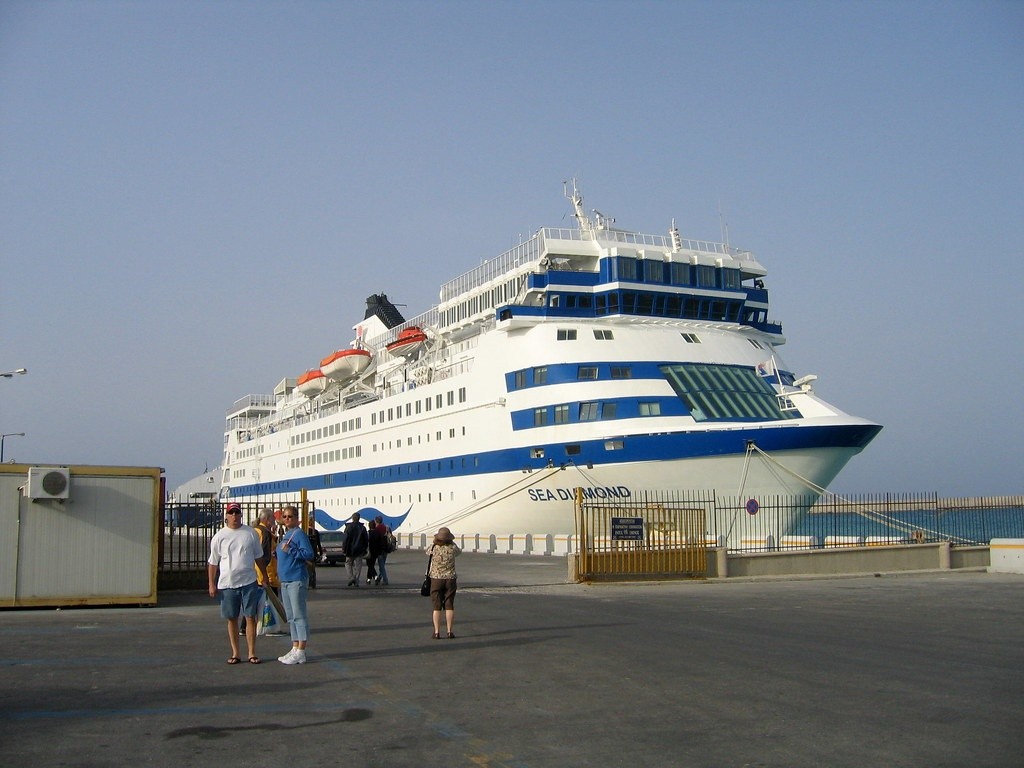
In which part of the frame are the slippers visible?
[249,656,261,664]
[227,658,241,664]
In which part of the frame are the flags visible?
[755,357,774,377]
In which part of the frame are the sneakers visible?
[277,645,306,664]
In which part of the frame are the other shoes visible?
[366,580,371,585]
[238,628,246,636]
[377,577,381,582]
[383,578,388,585]
[266,631,287,636]
[374,576,380,586]
[348,579,359,587]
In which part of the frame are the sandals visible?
[447,632,456,638]
[432,633,440,639]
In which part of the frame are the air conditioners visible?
[19,466,70,503]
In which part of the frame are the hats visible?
[434,527,455,540]
[226,503,241,512]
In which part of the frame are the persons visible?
[424,527,462,640]
[207,502,314,666]
[342,512,392,587]
[308,517,324,589]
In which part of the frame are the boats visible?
[384,326,427,359]
[165,467,224,529]
[319,347,372,381]
[296,369,327,396]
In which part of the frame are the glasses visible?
[282,515,296,518]
[227,511,241,514]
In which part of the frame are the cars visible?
[316,531,347,564]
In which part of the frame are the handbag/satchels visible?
[361,531,371,559]
[421,578,431,596]
[383,525,396,553]
[256,589,290,637]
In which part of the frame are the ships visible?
[223,180,884,554]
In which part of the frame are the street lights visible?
[1,433,25,462]
